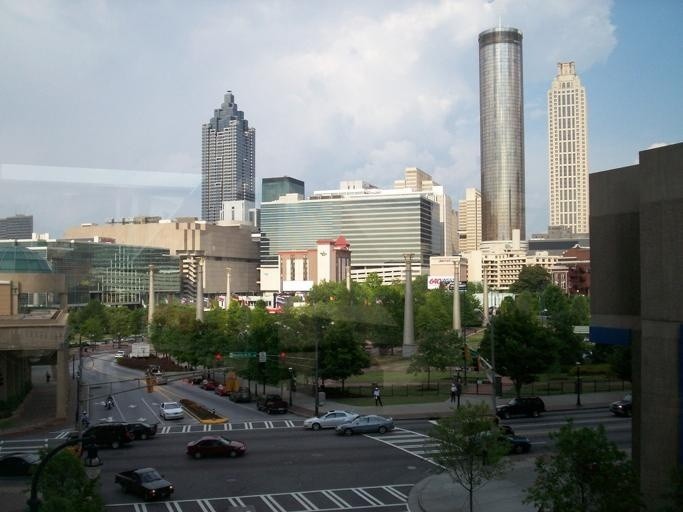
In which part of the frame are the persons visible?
[80,410,89,428]
[374,387,383,406]
[104,394,115,407]
[455,380,461,396]
[450,383,457,403]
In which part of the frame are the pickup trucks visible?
[114,466,173,501]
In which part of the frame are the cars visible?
[159,402,186,421]
[81,421,157,448]
[114,350,124,357]
[608,391,633,417]
[303,409,394,435]
[185,434,247,459]
[462,396,545,456]
[189,375,289,414]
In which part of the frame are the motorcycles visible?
[106,399,112,409]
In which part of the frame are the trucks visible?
[144,364,166,385]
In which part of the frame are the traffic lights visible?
[279,352,286,369]
[215,353,222,367]
